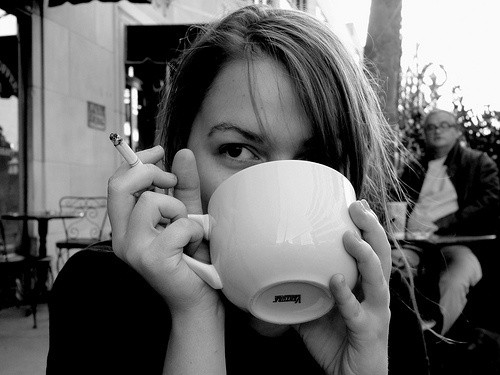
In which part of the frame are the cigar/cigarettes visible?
[110,132,158,193]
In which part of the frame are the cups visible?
[182,160,362,324]
[386,202,407,240]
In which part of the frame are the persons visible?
[45,5,464,375]
[379,110,500,337]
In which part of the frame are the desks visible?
[1,212,84,317]
[387,235,497,375]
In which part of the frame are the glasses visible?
[427,122,456,131]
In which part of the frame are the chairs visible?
[55,196,109,275]
[0,220,38,329]
